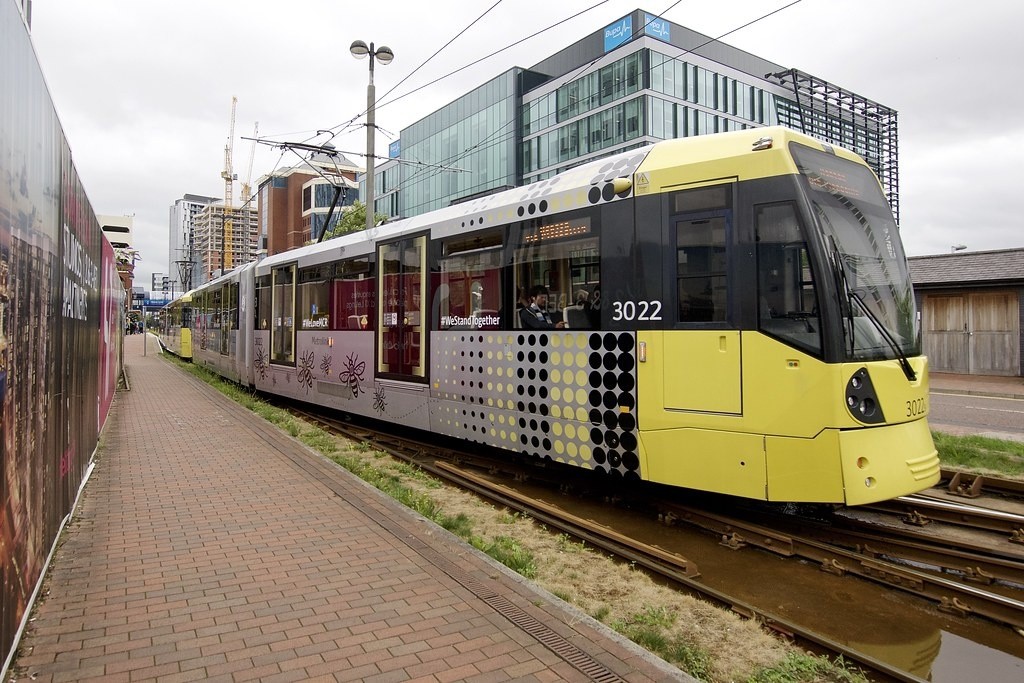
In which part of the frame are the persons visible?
[126,319,143,336]
[499,284,568,330]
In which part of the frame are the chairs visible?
[360,315,368,322]
[471,309,498,329]
[348,315,361,330]
[563,305,589,329]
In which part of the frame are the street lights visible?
[349,37,395,230]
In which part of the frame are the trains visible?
[156,124,945,509]
